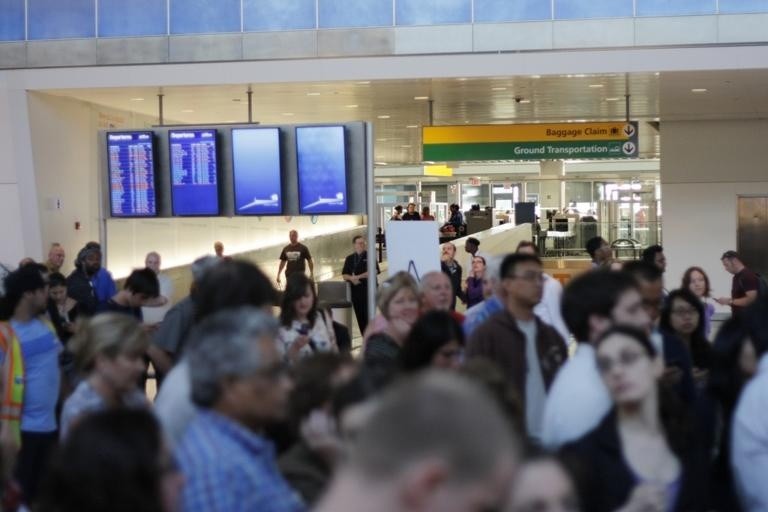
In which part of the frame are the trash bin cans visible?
[317,281,353,347]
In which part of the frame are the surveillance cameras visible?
[513,96,523,103]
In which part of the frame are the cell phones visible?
[301,323,308,335]
[713,298,719,302]
[64,323,72,329]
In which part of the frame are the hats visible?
[192,260,293,316]
